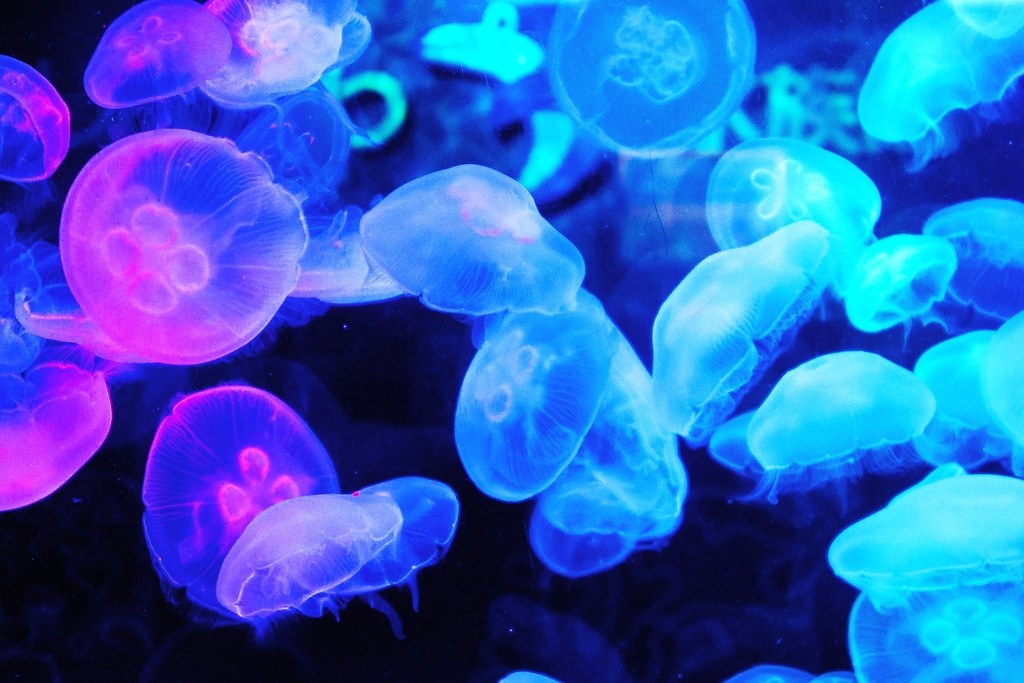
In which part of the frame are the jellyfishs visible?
[1,1,1023,682]
